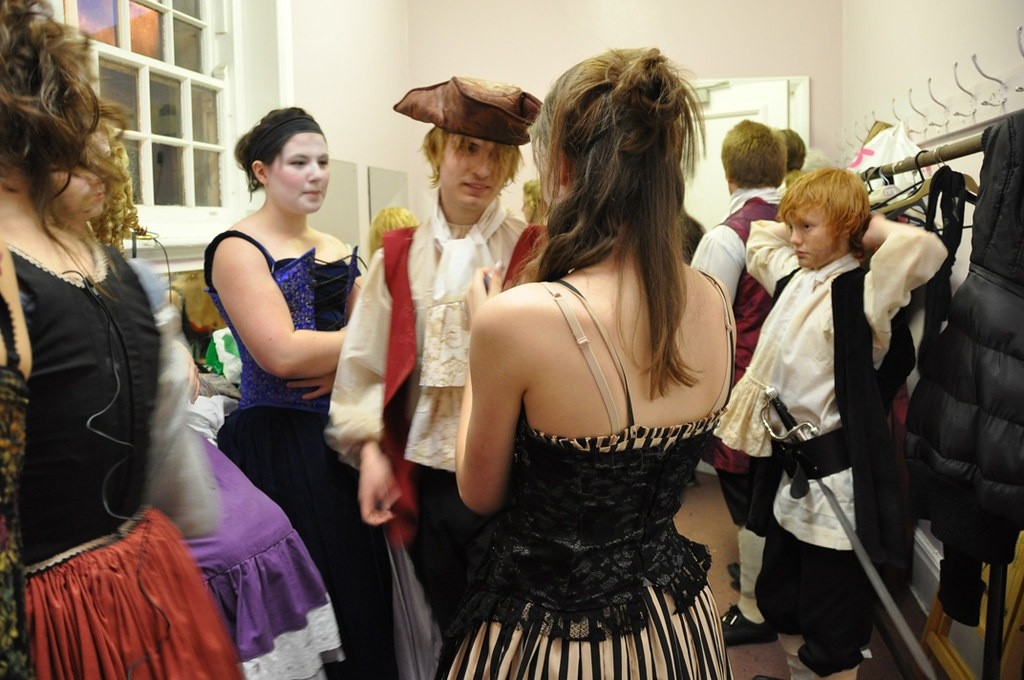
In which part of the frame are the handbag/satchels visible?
[143,304,223,536]
[206,326,243,385]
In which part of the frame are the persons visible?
[0,0,241,679]
[69,48,951,680]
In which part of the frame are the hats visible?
[391,77,543,145]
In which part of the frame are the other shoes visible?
[720,604,779,646]
[727,562,741,592]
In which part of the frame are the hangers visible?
[861,144,979,231]
